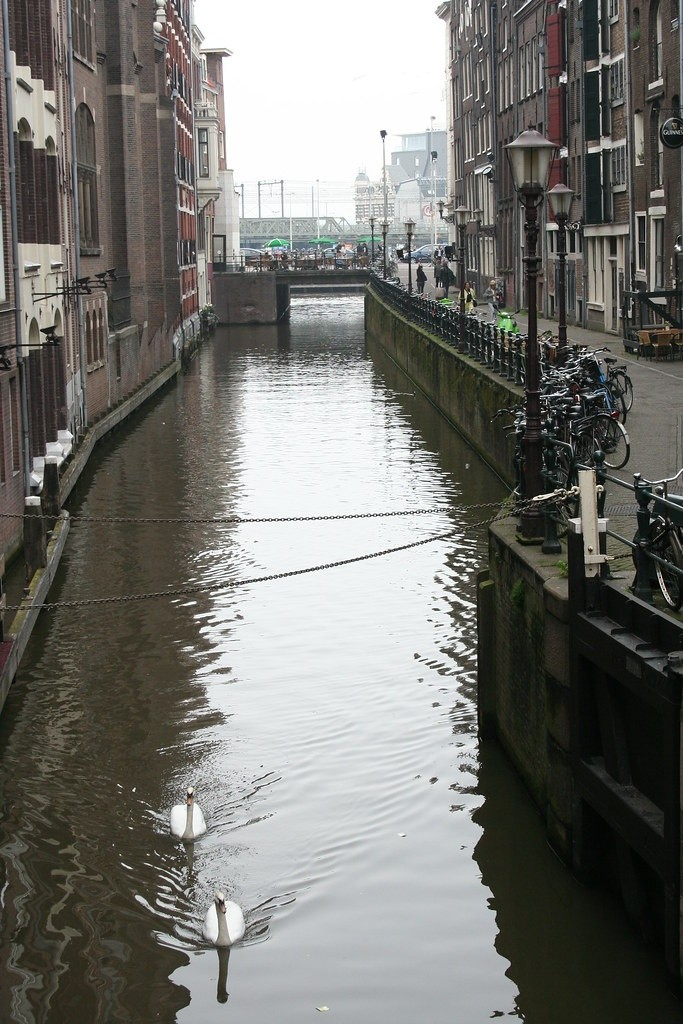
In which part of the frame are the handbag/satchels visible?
[473,300,477,307]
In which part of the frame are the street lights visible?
[431,150,437,240]
[403,217,416,294]
[380,129,387,221]
[368,213,377,267]
[545,180,576,352]
[381,219,391,281]
[453,204,472,354]
[501,122,559,546]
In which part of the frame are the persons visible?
[281,252,288,260]
[357,243,368,253]
[264,252,271,261]
[378,244,382,250]
[483,279,502,326]
[439,261,453,298]
[433,245,443,266]
[458,281,476,314]
[416,265,427,294]
[434,260,442,288]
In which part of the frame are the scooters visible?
[492,304,521,335]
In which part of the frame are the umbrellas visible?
[308,237,339,244]
[263,238,291,247]
[356,236,382,241]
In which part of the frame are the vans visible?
[403,244,455,263]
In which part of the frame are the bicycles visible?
[634,470,683,612]
[487,330,634,471]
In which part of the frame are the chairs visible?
[636,329,682,362]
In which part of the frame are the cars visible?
[240,248,270,267]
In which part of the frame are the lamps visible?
[56,273,109,290]
[0,324,61,373]
[435,199,455,225]
[473,207,497,239]
[32,276,91,299]
[487,151,496,163]
[72,267,119,283]
[483,167,496,183]
